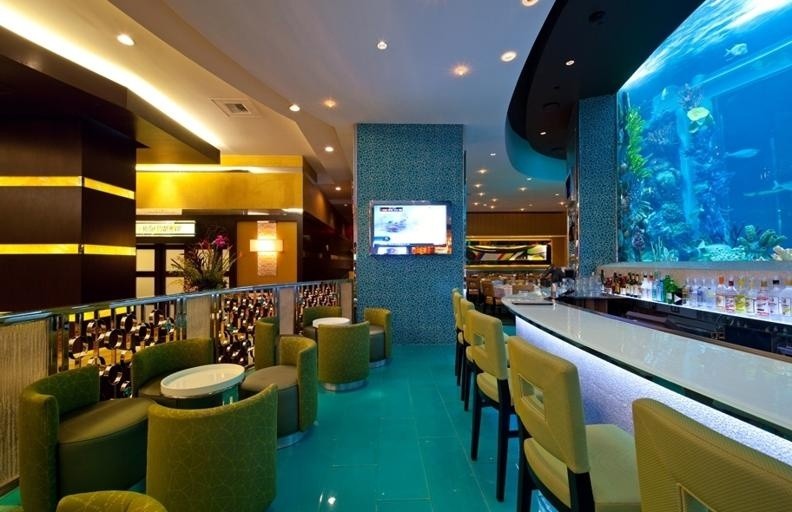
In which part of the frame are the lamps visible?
[248,239,283,252]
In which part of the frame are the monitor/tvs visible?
[369,200,453,256]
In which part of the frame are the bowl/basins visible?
[159,363,245,399]
[312,316,351,329]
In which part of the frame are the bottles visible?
[547,270,791,316]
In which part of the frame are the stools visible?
[632,397,790,512]
[468,310,544,503]
[453,289,510,410]
[509,336,639,512]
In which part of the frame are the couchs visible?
[56,491,165,510]
[132,338,221,409]
[145,384,277,510]
[253,304,390,392]
[240,336,318,450]
[16,365,149,510]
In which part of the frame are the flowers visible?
[168,232,243,292]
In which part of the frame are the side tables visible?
[160,362,245,408]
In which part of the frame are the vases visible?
[191,281,218,291]
